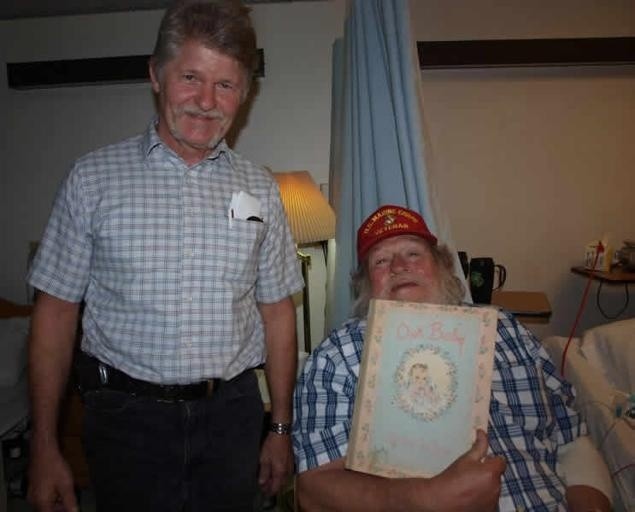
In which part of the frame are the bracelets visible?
[267,422,291,435]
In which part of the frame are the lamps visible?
[268,170,334,352]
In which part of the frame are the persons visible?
[290,207,615,511]
[27,4,306,511]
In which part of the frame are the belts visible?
[92,357,220,401]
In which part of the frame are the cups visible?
[469,257,506,305]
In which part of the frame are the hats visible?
[358,205,437,264]
